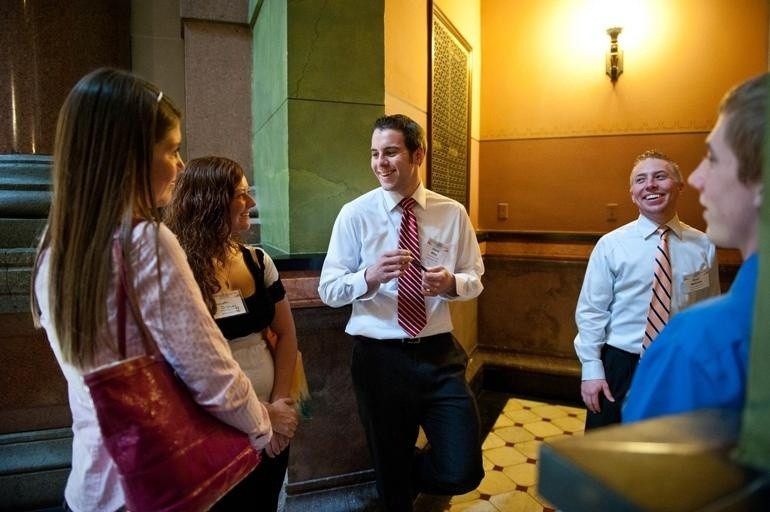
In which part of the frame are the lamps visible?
[605,26,627,90]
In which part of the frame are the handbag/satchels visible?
[246,246,315,424]
[82,218,263,512]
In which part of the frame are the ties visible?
[640,224,674,362]
[396,196,428,339]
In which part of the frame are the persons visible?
[317,114,485,512]
[164,157,309,512]
[622,72,770,425]
[30,68,272,512]
[573,150,722,430]
